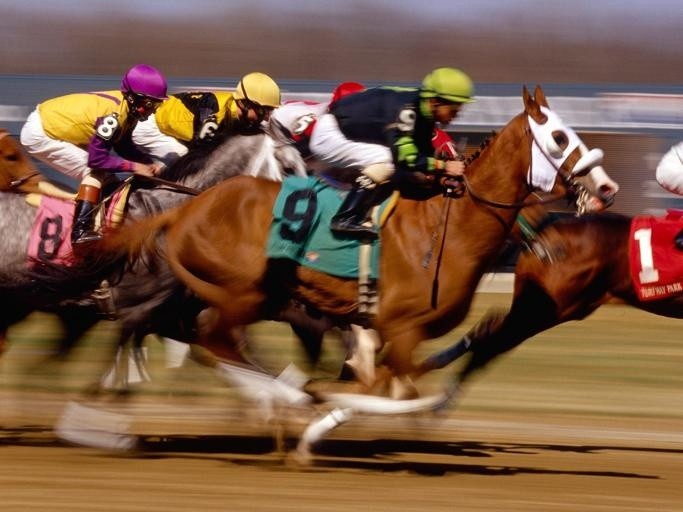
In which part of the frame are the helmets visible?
[120,62,170,101]
[231,72,282,108]
[421,66,476,105]
[332,79,366,102]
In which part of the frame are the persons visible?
[656,140,683,247]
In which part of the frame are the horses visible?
[70,81,619,467]
[407,207,682,413]
[0,117,310,393]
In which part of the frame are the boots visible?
[69,184,102,244]
[331,175,382,241]
